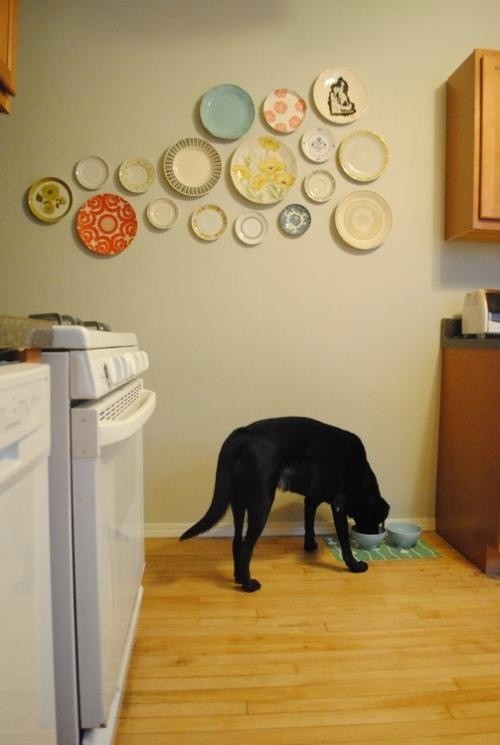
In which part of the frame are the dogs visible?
[179,417,390,594]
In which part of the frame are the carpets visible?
[322,533,440,563]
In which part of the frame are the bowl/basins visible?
[386,521,423,548]
[349,522,386,549]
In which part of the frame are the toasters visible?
[460,288,500,338]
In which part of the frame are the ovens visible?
[71,377,159,730]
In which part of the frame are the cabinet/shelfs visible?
[435,348,499,577]
[0,0,19,115]
[446,49,500,244]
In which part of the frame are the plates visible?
[159,137,222,196]
[144,194,187,230]
[229,64,394,252]
[73,155,111,190]
[190,202,235,238]
[75,193,136,255]
[198,85,256,138]
[117,156,156,194]
[28,178,76,224]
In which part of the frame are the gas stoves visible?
[16,307,149,400]
